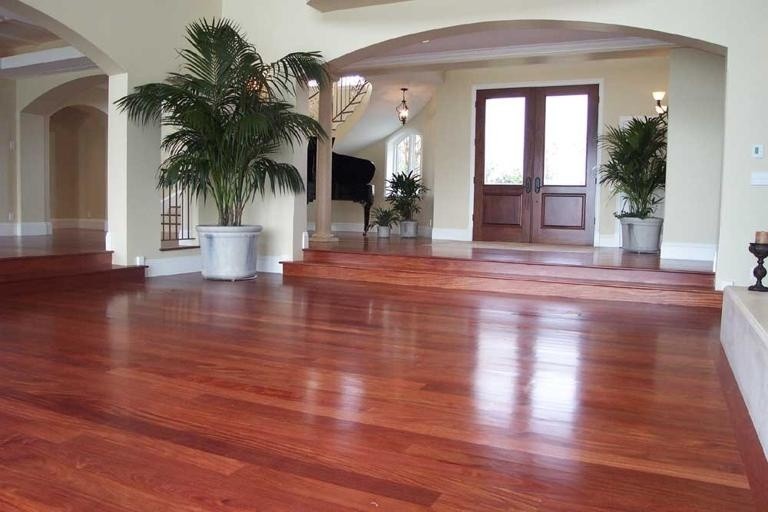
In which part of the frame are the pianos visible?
[306,147,375,235]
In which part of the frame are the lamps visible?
[396,88,409,127]
[652,90,668,126]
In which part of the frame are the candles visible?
[755,231,767,244]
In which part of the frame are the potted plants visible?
[366,206,401,239]
[385,169,432,238]
[592,111,669,253]
[112,15,333,281]
